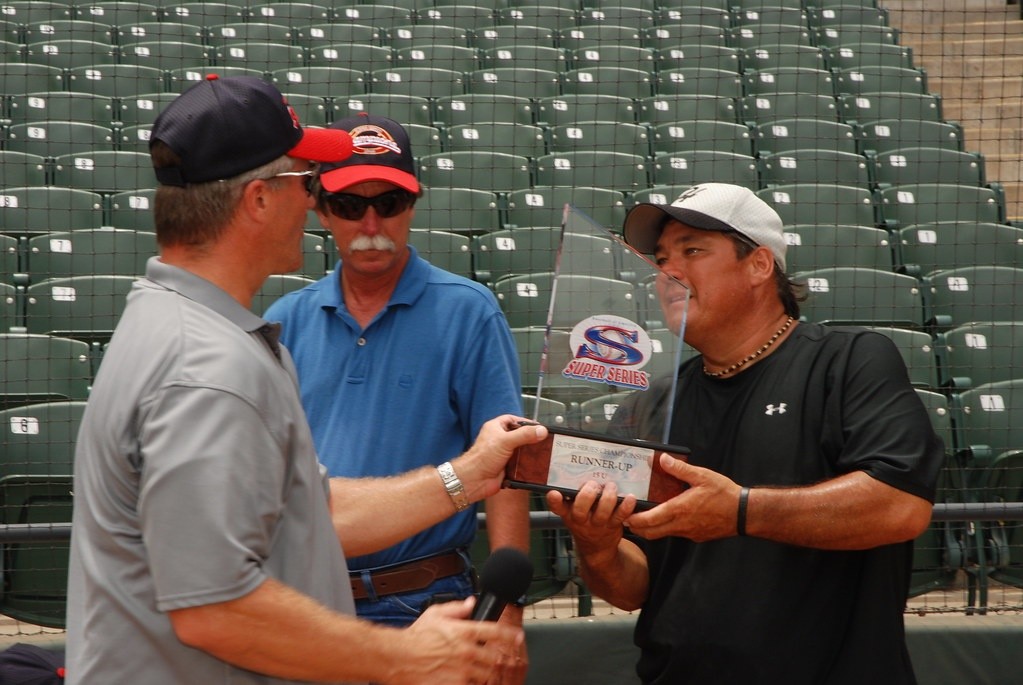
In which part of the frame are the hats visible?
[150,72,356,187]
[621,182,787,275]
[318,112,421,197]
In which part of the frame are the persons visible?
[65,76,548,685]
[544,182,940,685]
[259,111,530,684]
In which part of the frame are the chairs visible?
[0,0,1023,626]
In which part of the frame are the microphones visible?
[470,546,533,647]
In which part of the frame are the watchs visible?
[511,595,527,607]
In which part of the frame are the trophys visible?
[502,204,692,514]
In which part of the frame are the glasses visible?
[264,169,320,198]
[321,188,415,221]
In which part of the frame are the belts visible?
[348,551,475,599]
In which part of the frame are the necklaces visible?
[703,316,794,376]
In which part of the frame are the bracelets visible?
[438,462,471,512]
[736,487,749,536]
